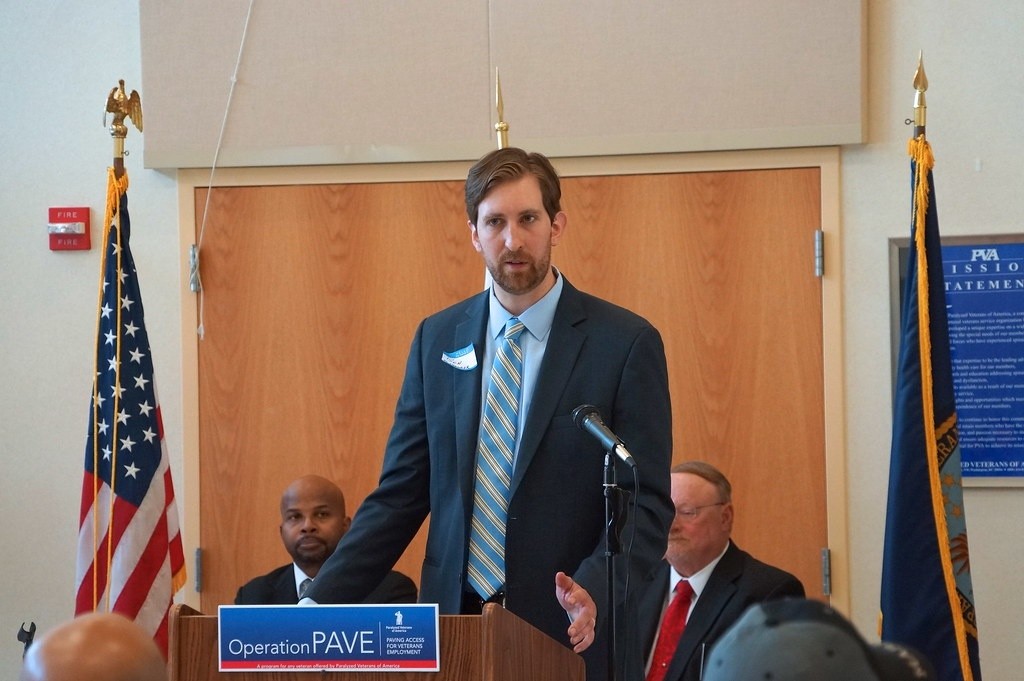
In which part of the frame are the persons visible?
[634,459,806,680]
[296,145,675,681]
[233,475,418,604]
[19,611,169,681]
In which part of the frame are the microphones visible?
[572,405,637,472]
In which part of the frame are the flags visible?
[873,142,984,681]
[74,168,187,665]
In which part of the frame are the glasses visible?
[674,502,725,520]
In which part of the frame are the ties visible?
[645,581,693,681]
[465,319,527,601]
[299,579,312,598]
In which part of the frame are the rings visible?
[592,617,596,627]
